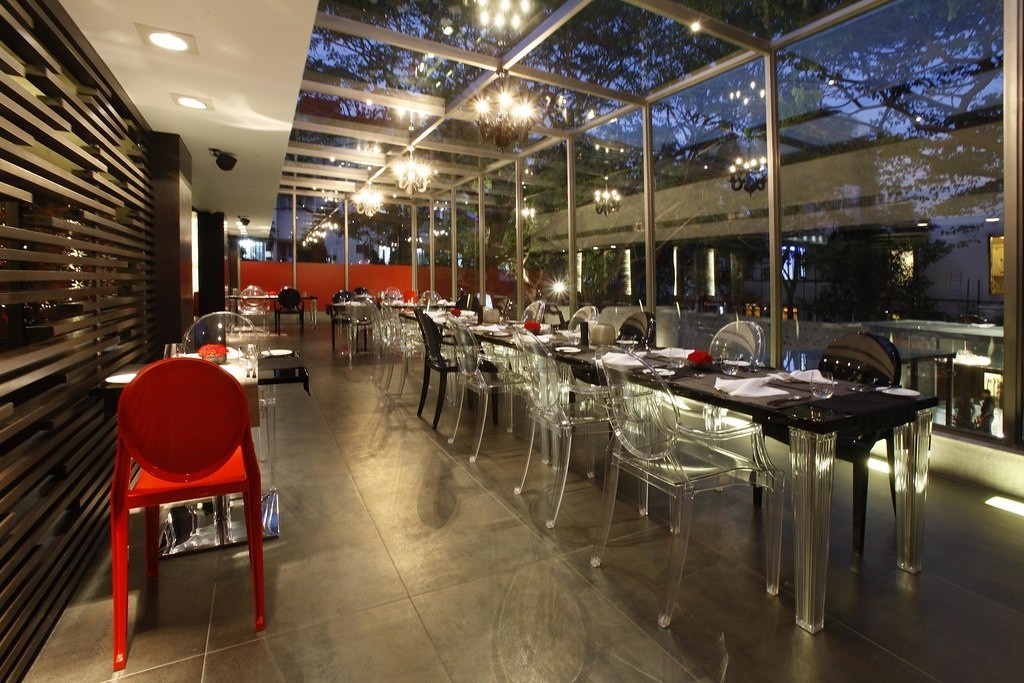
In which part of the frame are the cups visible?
[237,344,256,369]
[568,328,580,345]
[163,344,185,360]
[670,346,684,369]
[621,334,635,354]
[720,350,740,375]
[499,315,510,328]
[810,371,834,398]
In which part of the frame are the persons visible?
[979,390,994,433]
[954,392,975,429]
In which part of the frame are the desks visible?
[892,343,956,426]
[864,319,1005,397]
[401,310,940,633]
[108,363,281,559]
[228,294,318,329]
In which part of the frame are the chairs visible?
[587,342,785,630]
[677,319,763,493]
[326,286,656,530]
[273,286,306,336]
[107,358,267,672]
[180,311,260,457]
[240,285,271,328]
[753,331,902,552]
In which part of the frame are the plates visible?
[522,335,549,343]
[643,368,675,376]
[260,349,292,357]
[104,374,137,383]
[723,360,751,366]
[876,386,921,397]
[555,346,581,353]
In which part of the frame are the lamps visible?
[728,140,768,195]
[348,177,386,216]
[480,68,551,152]
[593,177,620,217]
[387,139,436,197]
